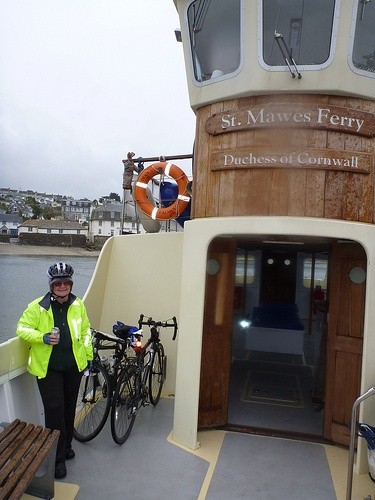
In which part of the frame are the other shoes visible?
[54,460,66,478]
[65,449,75,459]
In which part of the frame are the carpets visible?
[255,350,302,366]
[239,369,305,408]
[232,349,250,361]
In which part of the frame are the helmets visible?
[47,262,74,278]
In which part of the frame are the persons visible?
[16,261,94,481]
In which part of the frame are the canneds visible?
[51,327,60,343]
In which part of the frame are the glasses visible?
[53,282,71,287]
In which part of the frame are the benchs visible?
[0,418,60,500]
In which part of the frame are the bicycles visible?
[72,327,139,443]
[112,313,178,445]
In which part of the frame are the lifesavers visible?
[132,161,191,221]
